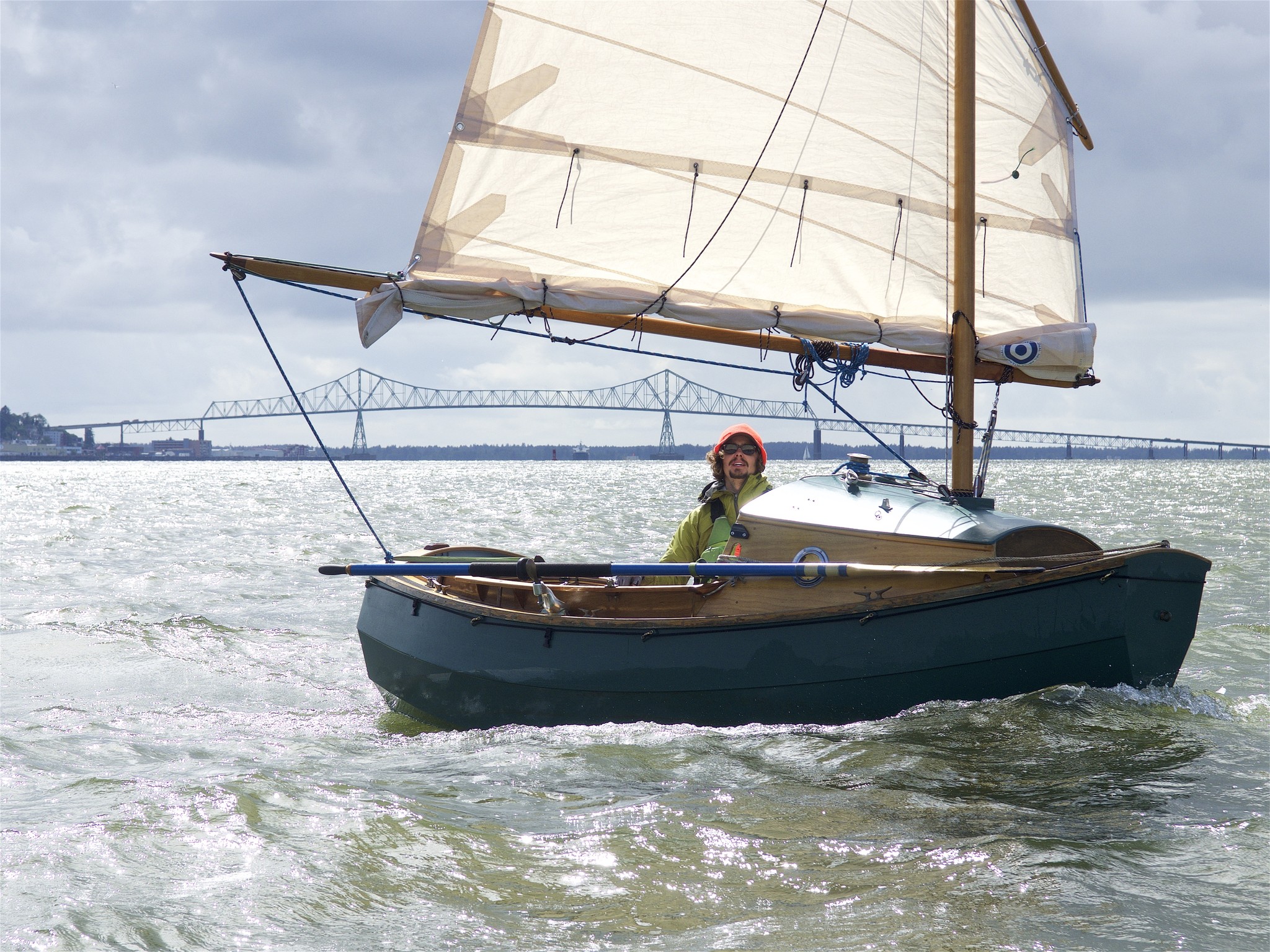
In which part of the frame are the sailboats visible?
[802,444,812,462]
[204,0,1212,745]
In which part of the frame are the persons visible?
[616,423,774,585]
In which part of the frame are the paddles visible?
[317,562,1047,578]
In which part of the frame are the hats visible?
[714,425,767,465]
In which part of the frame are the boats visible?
[572,440,590,460]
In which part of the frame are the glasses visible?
[723,444,757,456]
